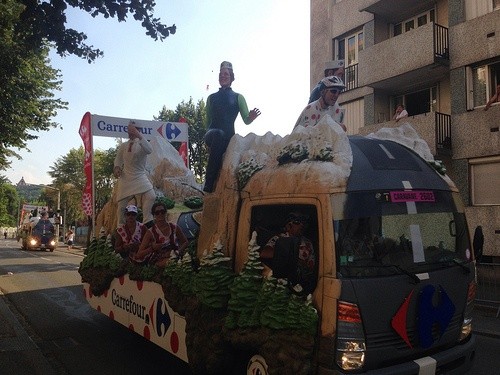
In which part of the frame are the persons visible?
[291,75,347,136]
[113,205,149,260]
[65,229,75,254]
[342,214,380,257]
[259,209,316,268]
[483,86,500,111]
[309,59,345,107]
[112,118,156,229]
[136,201,189,267]
[202,60,261,196]
[392,104,408,122]
[24,205,54,223]
[4,229,8,239]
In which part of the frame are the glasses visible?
[288,219,305,225]
[153,209,167,216]
[126,213,137,217]
[328,90,343,95]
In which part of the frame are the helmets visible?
[320,76,346,90]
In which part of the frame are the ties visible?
[128,142,134,152]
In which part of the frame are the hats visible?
[220,61,232,70]
[287,211,306,221]
[125,205,138,213]
[324,60,344,70]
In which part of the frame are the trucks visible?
[81,134,486,375]
[16,219,58,252]
